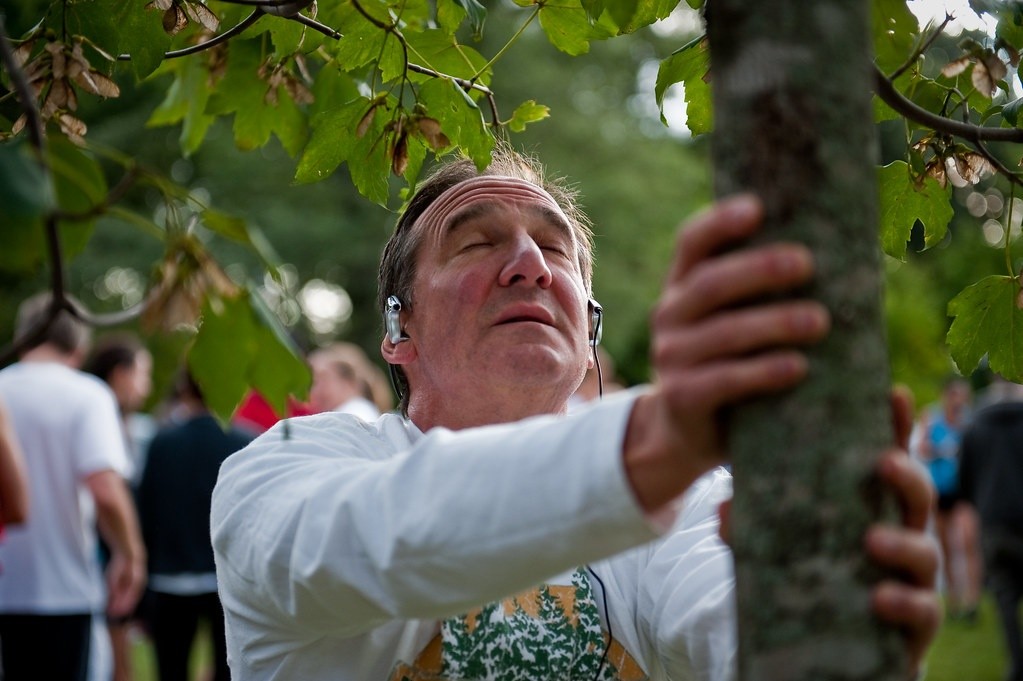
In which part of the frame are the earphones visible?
[384,294,411,345]
[587,298,604,349]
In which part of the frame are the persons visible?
[955,379,1023,677]
[306,345,395,425]
[141,372,254,681]
[82,334,154,681]
[0,294,149,681]
[94,409,154,681]
[909,368,984,626]
[569,347,627,408]
[211,151,939,681]
[0,398,28,531]
